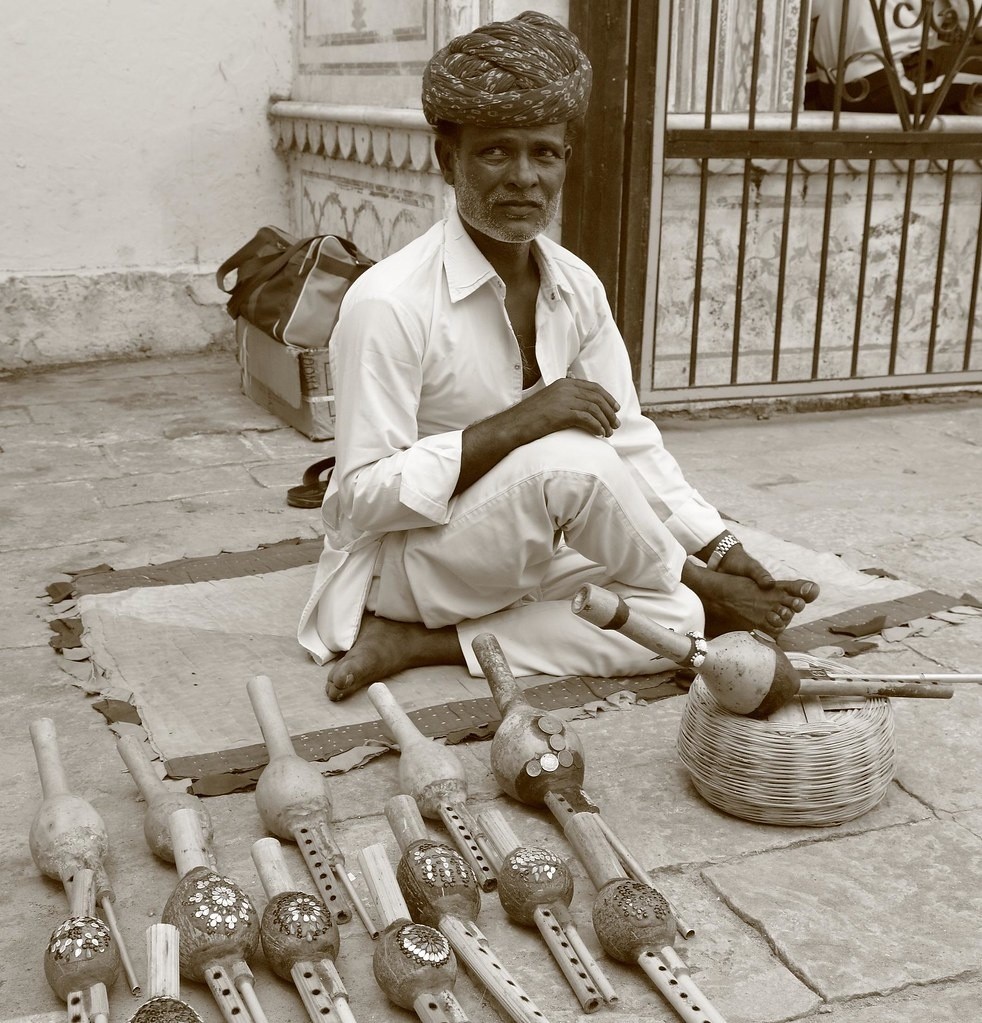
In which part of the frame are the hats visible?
[420,10,593,126]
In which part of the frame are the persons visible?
[298,8,820,706]
[809,0,982,117]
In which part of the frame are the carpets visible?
[38,509,982,799]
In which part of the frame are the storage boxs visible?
[234,316,336,441]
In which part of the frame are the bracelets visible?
[704,533,740,572]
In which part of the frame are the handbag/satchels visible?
[215,223,377,347]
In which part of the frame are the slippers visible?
[286,456,335,508]
